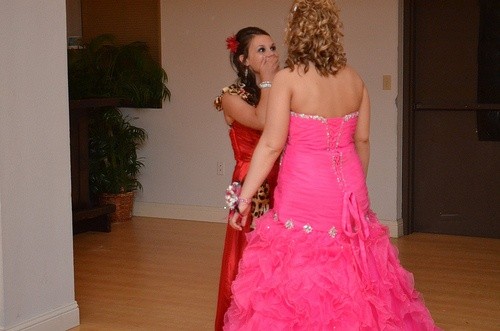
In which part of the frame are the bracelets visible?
[237,198,254,206]
[256,81,273,87]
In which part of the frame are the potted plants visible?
[89,106,149,224]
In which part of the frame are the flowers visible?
[226,32,239,54]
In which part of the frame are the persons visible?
[222,0,446,331]
[211,25,280,331]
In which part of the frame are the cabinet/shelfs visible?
[67,97,121,235]
